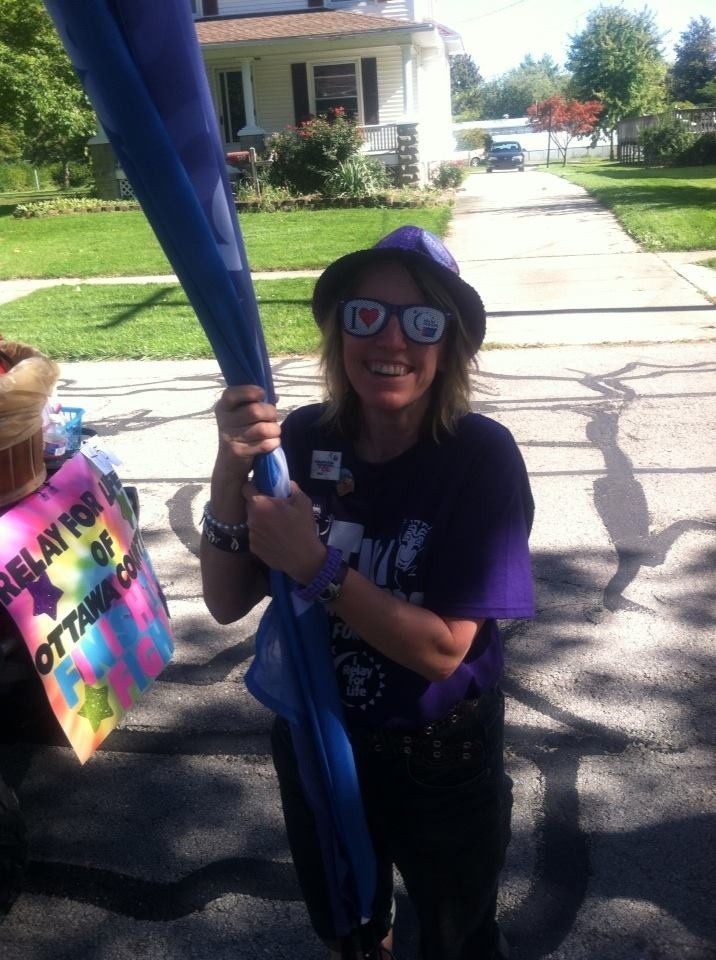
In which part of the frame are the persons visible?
[201,223,539,960]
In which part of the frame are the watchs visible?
[316,560,347,602]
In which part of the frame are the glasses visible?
[335,296,451,346]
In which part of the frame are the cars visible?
[484,140,528,173]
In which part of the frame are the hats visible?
[311,225,487,363]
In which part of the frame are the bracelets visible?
[201,518,252,555]
[293,545,343,602]
[200,500,250,533]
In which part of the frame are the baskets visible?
[44,406,86,469]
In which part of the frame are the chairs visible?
[511,146,516,152]
[495,148,501,152]
[503,148,508,151]
